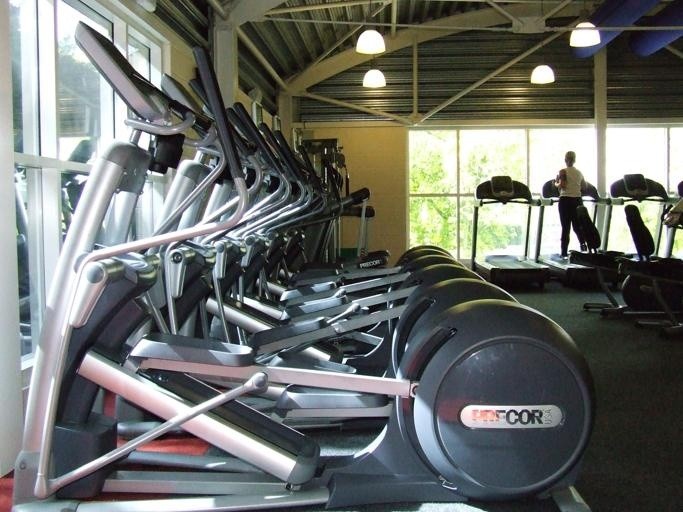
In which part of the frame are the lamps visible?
[356,15,385,56]
[531,62,555,85]
[570,10,601,47]
[362,59,387,88]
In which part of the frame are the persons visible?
[554,151,588,260]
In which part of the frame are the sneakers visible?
[579,245,587,254]
[559,255,569,260]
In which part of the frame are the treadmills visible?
[471,176,551,288]
[598,174,683,275]
[534,178,613,284]
